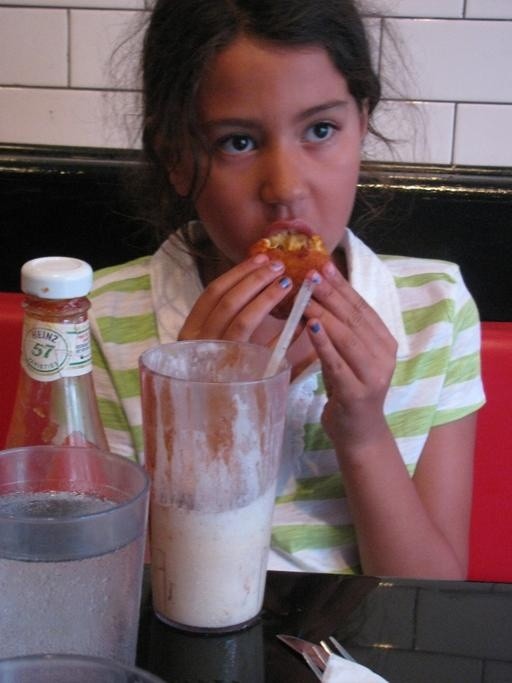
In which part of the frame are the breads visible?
[245,231,330,318]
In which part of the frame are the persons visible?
[50,0,488,582]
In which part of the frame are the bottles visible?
[2,256,109,448]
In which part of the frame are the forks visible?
[302,637,356,683]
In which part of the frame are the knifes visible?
[276,634,325,668]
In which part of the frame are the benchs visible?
[1,292,512,585]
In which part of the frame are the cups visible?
[0,655,160,683]
[1,446,148,662]
[138,339,293,635]
